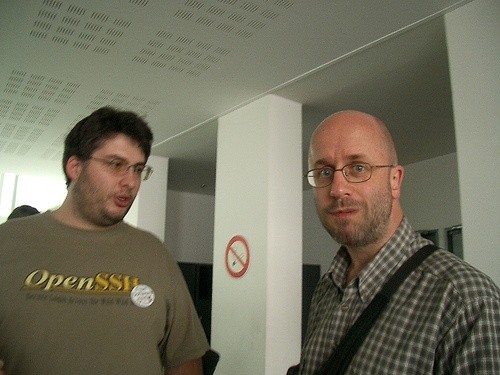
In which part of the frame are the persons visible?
[1,105,212,375]
[297,110,500,374]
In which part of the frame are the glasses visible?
[82,154,154,182]
[303,162,395,189]
